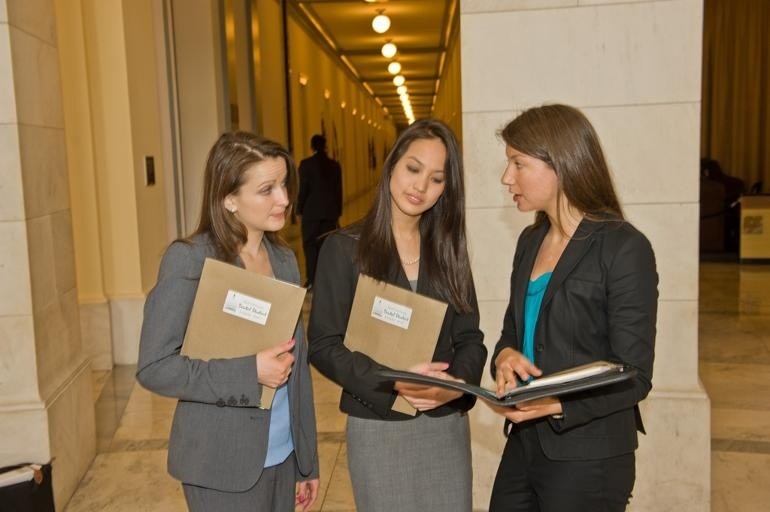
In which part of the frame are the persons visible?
[304,118,488,512]
[295,134,344,294]
[490,103,659,510]
[133,130,320,512]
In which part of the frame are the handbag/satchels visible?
[0,463,54,512]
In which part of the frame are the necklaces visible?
[398,256,421,266]
